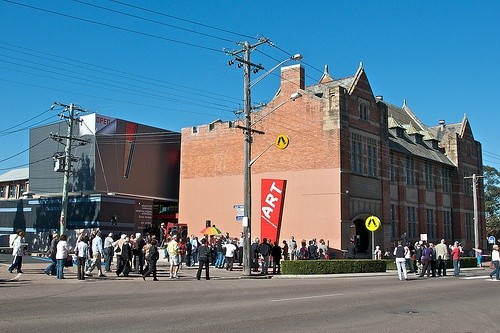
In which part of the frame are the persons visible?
[166,235,180,279]
[8,229,28,273]
[348,237,357,259]
[44,228,330,282]
[222,239,236,271]
[373,238,463,278]
[196,238,210,280]
[490,244,500,280]
[56,235,71,279]
[142,239,159,281]
[472,247,482,267]
[393,239,408,281]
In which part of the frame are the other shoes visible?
[44,271,49,275]
[154,278,158,281]
[173,275,179,278]
[9,268,12,273]
[142,275,145,280]
[169,275,173,278]
[17,270,22,273]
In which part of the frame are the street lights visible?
[241,51,304,275]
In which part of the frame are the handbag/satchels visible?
[75,247,78,255]
[123,261,129,274]
[421,256,426,263]
[65,255,72,267]
[46,250,53,258]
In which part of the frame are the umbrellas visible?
[200,226,222,235]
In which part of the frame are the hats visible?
[53,233,58,238]
